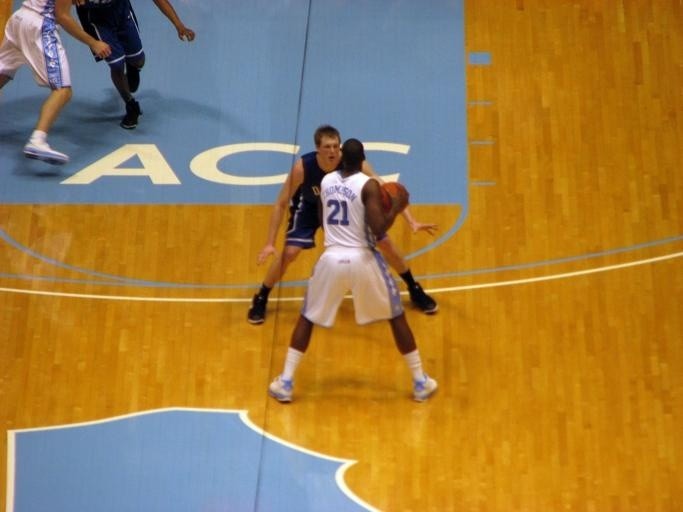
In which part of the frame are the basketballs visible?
[380,182,408,213]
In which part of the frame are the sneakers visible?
[126,66,140,92]
[247,294,268,324]
[269,375,293,402]
[407,282,438,313]
[414,374,439,402]
[120,101,143,129]
[23,139,69,166]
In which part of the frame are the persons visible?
[0,0,195,165]
[247,126,437,323]
[269,137,437,402]
